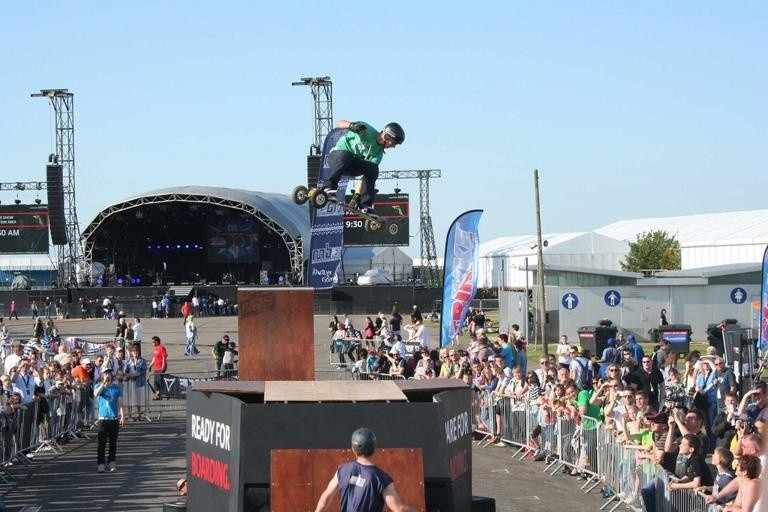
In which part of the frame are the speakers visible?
[47,165,67,245]
[307,155,322,225]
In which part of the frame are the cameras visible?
[740,418,756,434]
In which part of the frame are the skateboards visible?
[290,185,385,231]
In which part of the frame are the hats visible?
[646,411,669,423]
[102,367,113,373]
[116,347,123,352]
[369,347,375,353]
[82,358,91,366]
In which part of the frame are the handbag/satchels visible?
[694,387,708,410]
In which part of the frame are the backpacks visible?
[572,358,593,391]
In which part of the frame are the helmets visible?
[351,429,376,453]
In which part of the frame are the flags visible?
[441,210,483,348]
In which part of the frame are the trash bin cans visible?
[659,324,693,358]
[577,326,618,358]
[707,324,758,367]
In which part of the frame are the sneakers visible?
[472,433,599,483]
[601,484,641,508]
[98,464,117,473]
[1,432,81,470]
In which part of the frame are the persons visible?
[149,336,168,401]
[212,335,239,381]
[151,291,239,319]
[2,295,144,356]
[710,321,727,339]
[1,344,147,468]
[93,368,125,472]
[659,309,669,326]
[328,302,528,448]
[312,428,416,512]
[523,334,767,512]
[322,117,406,218]
[184,314,200,356]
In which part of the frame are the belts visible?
[99,417,118,421]
